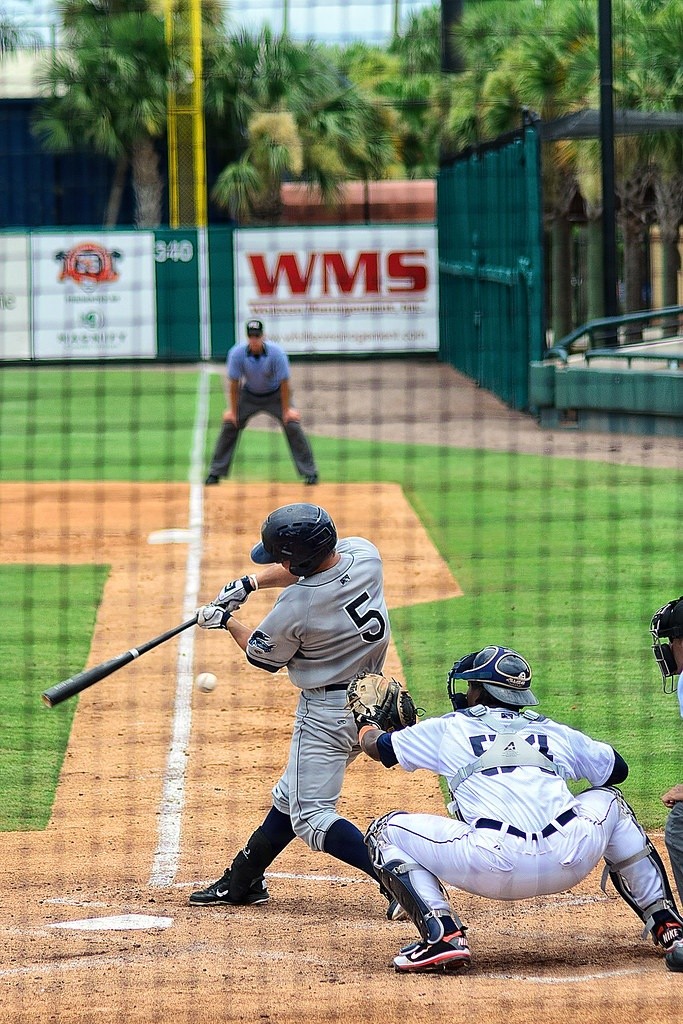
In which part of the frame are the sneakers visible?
[386,893,406,920]
[392,924,471,973]
[189,868,270,907]
[654,922,683,973]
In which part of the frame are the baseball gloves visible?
[345,673,420,735]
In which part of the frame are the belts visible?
[475,806,575,840]
[324,672,383,691]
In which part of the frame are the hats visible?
[247,321,261,335]
[668,599,683,638]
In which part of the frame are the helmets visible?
[473,646,540,706]
[251,504,337,576]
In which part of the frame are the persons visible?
[189,502,405,920]
[649,596,683,906]
[347,644,683,972]
[206,320,319,486]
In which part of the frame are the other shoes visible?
[306,474,318,485]
[206,474,217,484]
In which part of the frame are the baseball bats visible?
[42,616,198,709]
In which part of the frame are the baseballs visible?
[195,671,218,694]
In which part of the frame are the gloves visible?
[196,604,233,631]
[213,576,257,612]
